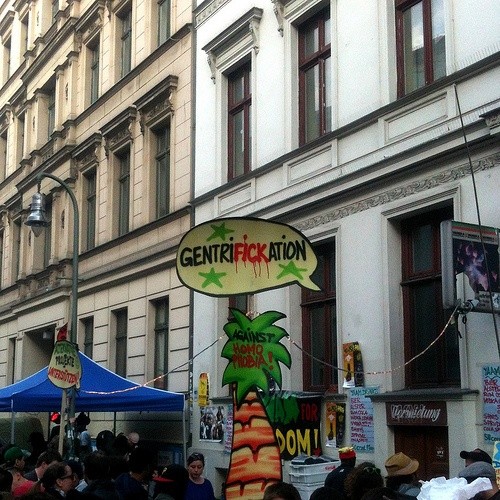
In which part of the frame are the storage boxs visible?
[292,483,324,500]
[288,461,341,483]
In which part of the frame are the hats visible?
[187,452,204,462]
[460,448,492,465]
[337,446,357,460]
[4,447,32,460]
[42,463,57,487]
[153,464,189,483]
[126,455,145,473]
[384,452,419,477]
[458,461,496,478]
[399,483,420,497]
[25,431,48,443]
[126,432,140,444]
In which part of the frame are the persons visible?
[0,413,217,500]
[200,408,224,441]
[263,446,500,500]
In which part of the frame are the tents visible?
[0,344,187,469]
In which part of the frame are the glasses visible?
[362,467,382,476]
[60,472,74,481]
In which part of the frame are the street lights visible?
[22,172,79,458]
